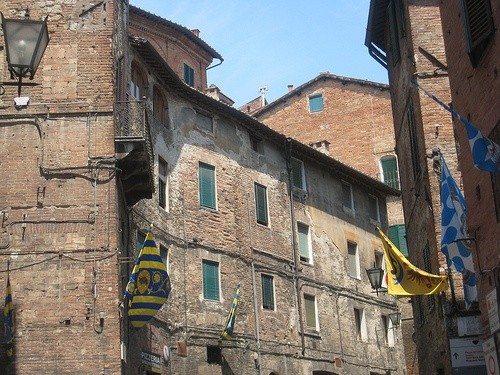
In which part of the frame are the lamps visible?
[1,6,52,95]
[365,261,384,292]
[387,306,401,329]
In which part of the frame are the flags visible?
[2,261,15,362]
[437,150,476,310]
[122,226,174,332]
[218,283,241,345]
[377,228,449,295]
[410,80,500,175]
